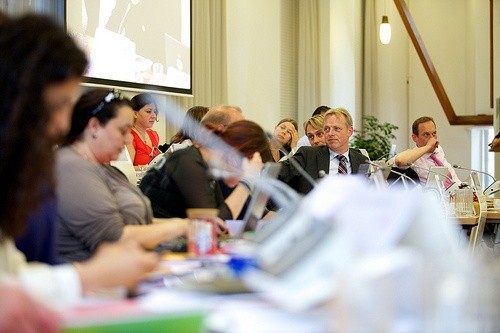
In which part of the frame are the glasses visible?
[92,88,124,115]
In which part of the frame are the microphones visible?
[365,160,418,186]
[453,165,496,182]
[266,132,318,189]
[407,162,454,183]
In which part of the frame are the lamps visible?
[379,0,391,44]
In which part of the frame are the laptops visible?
[425,167,449,187]
[214,162,282,242]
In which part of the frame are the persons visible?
[0,11,500,332]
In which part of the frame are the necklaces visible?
[134,125,146,144]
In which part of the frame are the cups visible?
[223,219,246,239]
[493,199,500,209]
[454,189,475,217]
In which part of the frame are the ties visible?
[430,153,452,189]
[335,154,348,175]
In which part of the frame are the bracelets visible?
[239,177,253,193]
